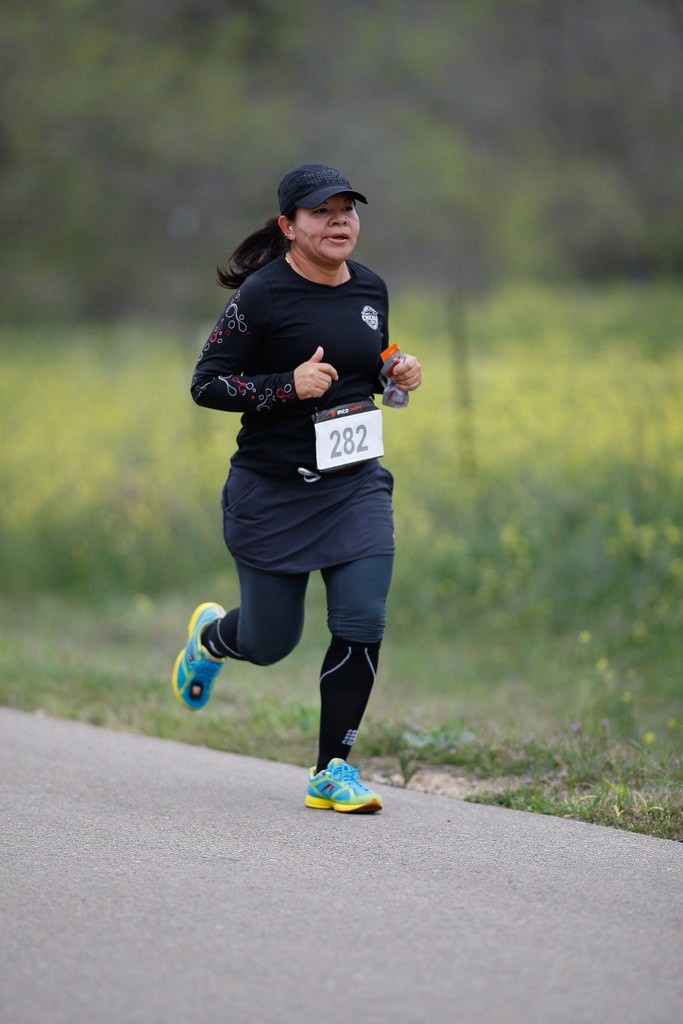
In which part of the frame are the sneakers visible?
[172,601,226,712]
[305,758,382,813]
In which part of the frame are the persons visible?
[169,168,418,816]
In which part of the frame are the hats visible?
[278,164,368,214]
